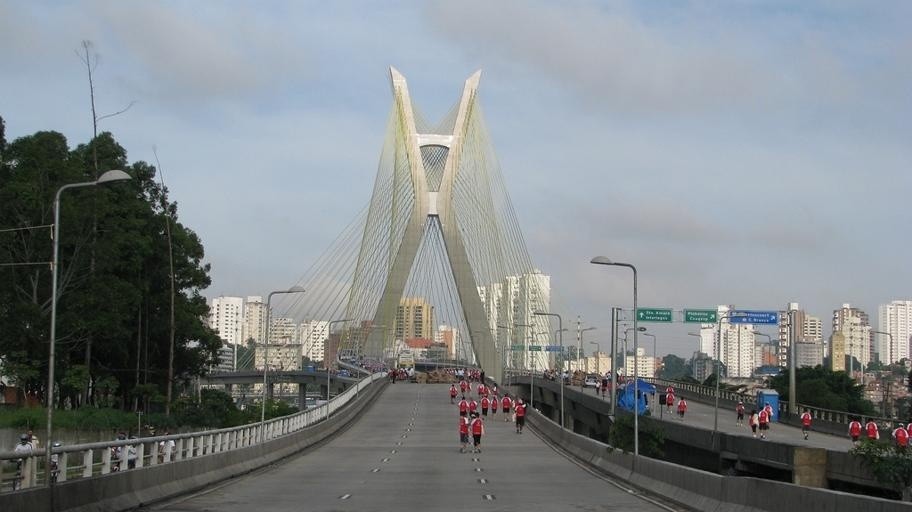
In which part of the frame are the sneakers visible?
[803,434,808,440]
[752,432,766,440]
[736,424,743,427]
[484,417,522,434]
[458,447,481,453]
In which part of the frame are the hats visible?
[898,422,904,426]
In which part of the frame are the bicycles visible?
[7,457,57,491]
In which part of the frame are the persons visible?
[749,410,759,437]
[764,402,773,430]
[800,408,812,440]
[446,368,526,453]
[678,397,687,418]
[11,429,61,474]
[666,385,674,393]
[758,407,769,438]
[865,418,878,444]
[848,417,862,445]
[109,428,177,467]
[891,423,909,453]
[907,418,912,447]
[595,371,656,402]
[666,391,674,413]
[388,366,414,383]
[736,401,745,426]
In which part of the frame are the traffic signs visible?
[636,308,672,323]
[682,309,718,323]
[728,309,777,325]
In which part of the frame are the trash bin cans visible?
[757,389,779,422]
[307,366,313,371]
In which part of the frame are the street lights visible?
[325,318,390,419]
[588,255,640,456]
[870,329,895,428]
[259,283,305,444]
[642,332,657,418]
[41,170,134,491]
[688,332,705,386]
[713,312,750,431]
[478,310,599,427]
[623,325,645,410]
[752,329,773,389]
